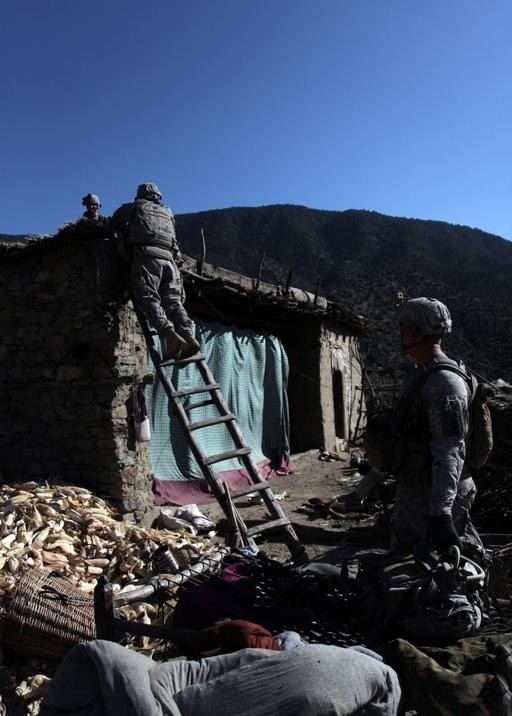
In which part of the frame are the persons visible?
[79,193,101,222]
[373,294,486,581]
[108,181,202,371]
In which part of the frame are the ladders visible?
[129,289,308,567]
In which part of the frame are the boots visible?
[162,327,199,366]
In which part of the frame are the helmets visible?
[134,183,162,201]
[398,297,452,335]
[82,194,100,206]
